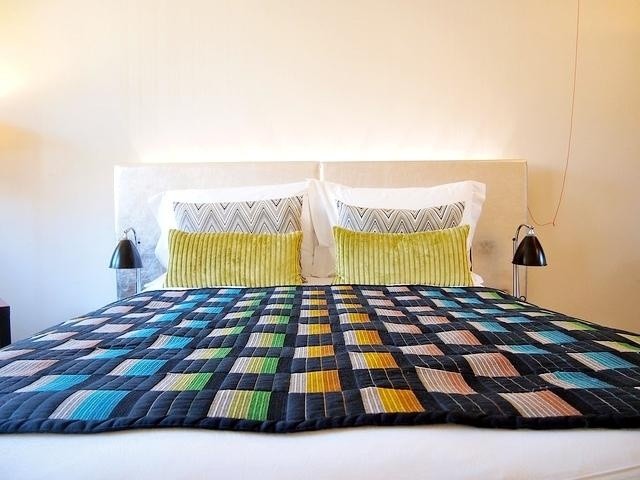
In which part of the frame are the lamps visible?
[512,223,547,298]
[108,227,143,293]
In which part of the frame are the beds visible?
[0,158,640,480]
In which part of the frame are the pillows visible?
[143,179,317,290]
[162,229,302,287]
[172,194,308,283]
[331,224,474,287]
[308,180,487,288]
[336,199,465,233]
